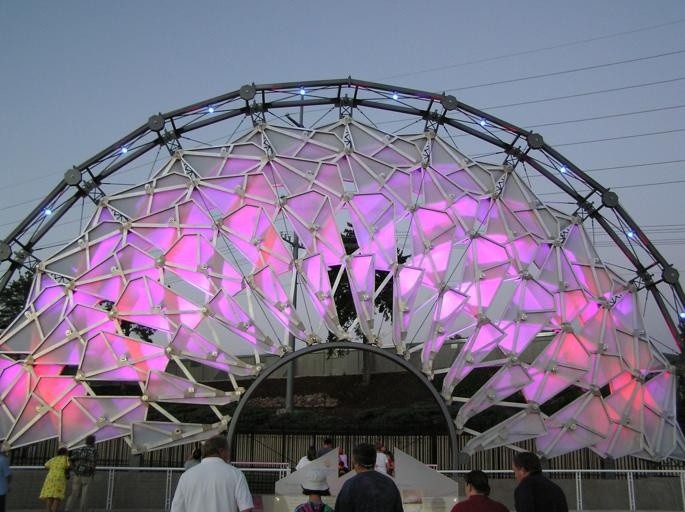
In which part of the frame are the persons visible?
[0,443,14,511]
[183,449,202,471]
[290,436,404,511]
[37,447,72,511]
[511,452,569,512]
[169,435,255,511]
[62,435,98,511]
[449,469,510,512]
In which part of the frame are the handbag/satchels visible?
[63,467,71,480]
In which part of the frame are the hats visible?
[302,468,330,491]
